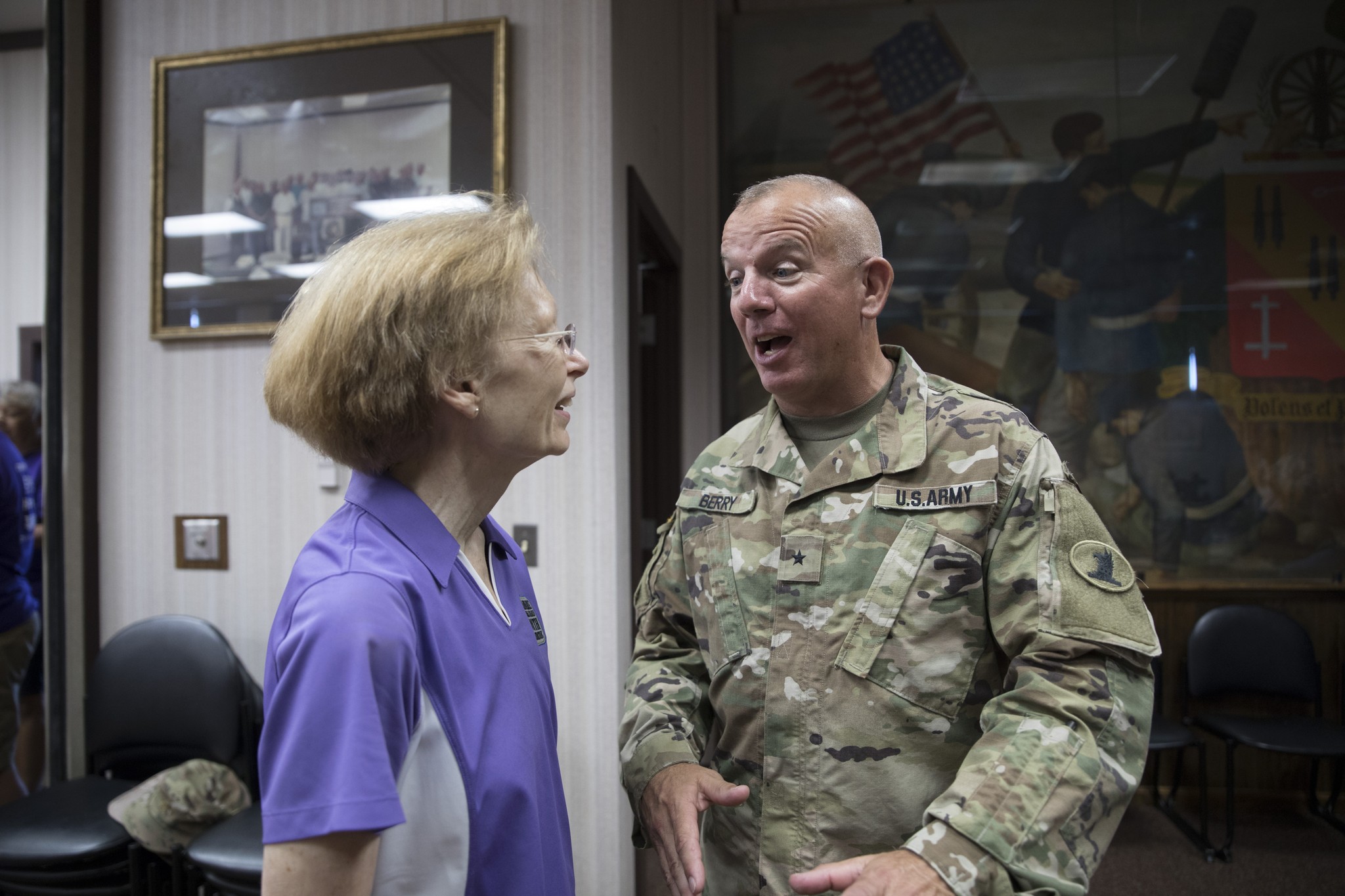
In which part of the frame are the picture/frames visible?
[711,0,1344,594]
[151,14,512,346]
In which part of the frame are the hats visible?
[109,757,252,855]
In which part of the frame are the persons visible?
[258,193,592,896]
[0,380,50,800]
[227,162,422,264]
[619,171,1161,896]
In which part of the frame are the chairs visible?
[0,614,263,895]
[1171,603,1345,862]
[1145,656,1217,865]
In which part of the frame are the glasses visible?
[491,323,579,358]
